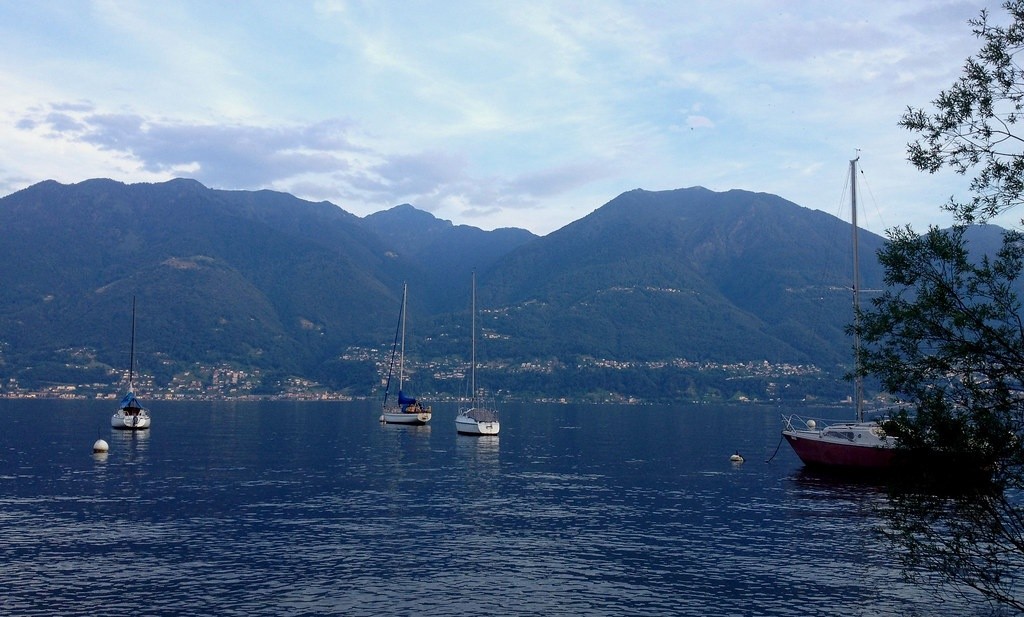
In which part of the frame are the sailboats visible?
[110,296,151,429]
[780,147,1017,476]
[379,280,432,425]
[454,265,500,435]
